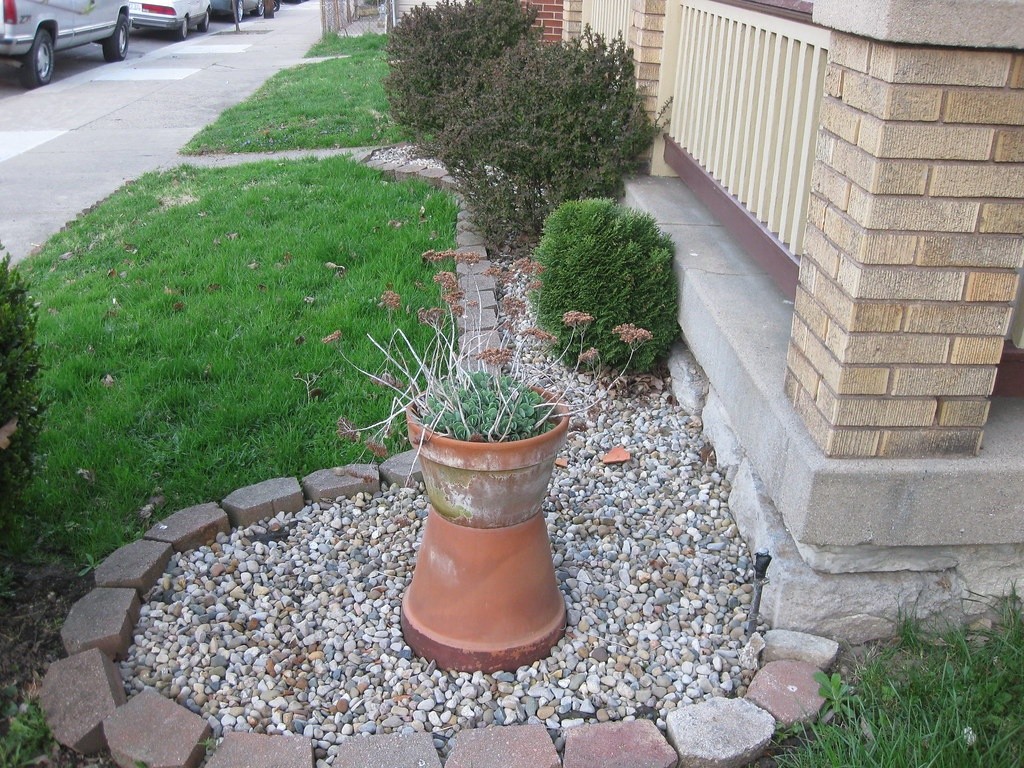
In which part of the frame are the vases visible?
[399,507,570,681]
[405,382,571,530]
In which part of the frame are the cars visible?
[124,0,214,41]
[211,0,264,23]
[0,0,131,90]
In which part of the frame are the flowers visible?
[319,246,649,526]
